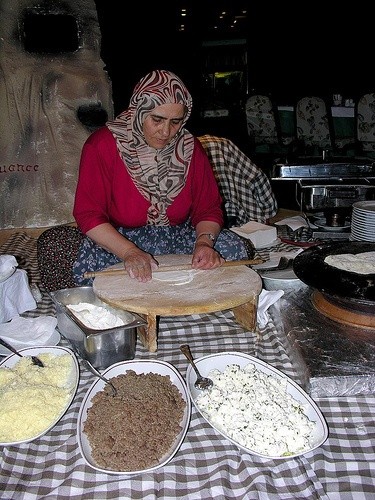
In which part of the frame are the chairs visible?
[294,95,337,158]
[244,95,283,161]
[354,91,375,152]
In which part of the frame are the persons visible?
[73,70,252,287]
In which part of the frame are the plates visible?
[0,323,60,356]
[348,200,375,242]
[249,252,300,282]
[314,219,350,231]
[0,346,79,446]
[76,359,190,474]
[186,351,328,460]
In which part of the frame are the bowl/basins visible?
[48,287,146,371]
[325,213,346,227]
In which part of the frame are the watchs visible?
[198,233,215,245]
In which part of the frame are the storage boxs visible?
[47,286,148,369]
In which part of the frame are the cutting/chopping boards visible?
[92,253,262,316]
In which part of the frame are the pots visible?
[291,241,375,303]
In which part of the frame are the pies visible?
[324,251,375,275]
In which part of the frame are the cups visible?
[334,100,341,106]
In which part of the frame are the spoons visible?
[180,345,213,391]
[82,360,116,396]
[254,257,294,273]
[0,338,43,367]
